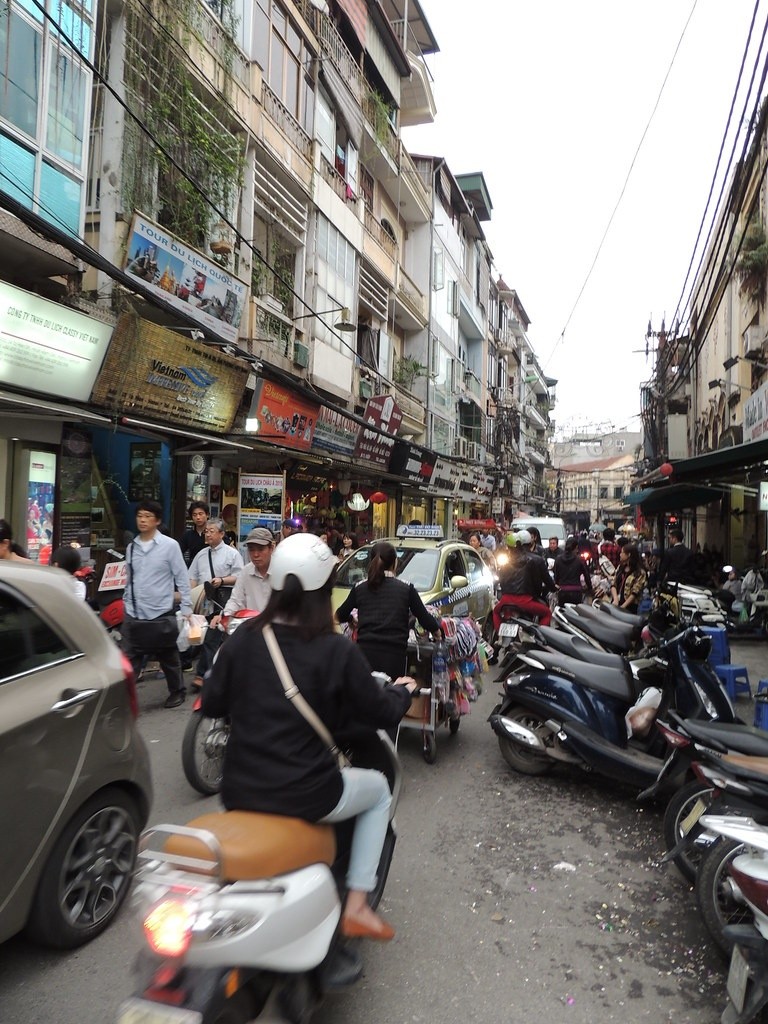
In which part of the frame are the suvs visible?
[0,556,154,951]
[115,675,432,1023]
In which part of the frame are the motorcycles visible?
[178,583,347,797]
[64,542,127,642]
[484,555,768,1024]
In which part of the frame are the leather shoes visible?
[164,691,184,708]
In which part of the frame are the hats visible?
[505,532,523,548]
[517,530,532,544]
[245,527,273,545]
[280,519,298,528]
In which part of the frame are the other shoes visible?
[182,662,193,672]
[340,913,396,939]
[157,670,165,679]
[191,677,203,687]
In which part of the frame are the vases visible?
[259,293,286,312]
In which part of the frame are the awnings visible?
[632,438,768,488]
[624,483,726,514]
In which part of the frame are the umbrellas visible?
[589,524,607,536]
[618,522,638,537]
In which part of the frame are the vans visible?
[510,516,566,551]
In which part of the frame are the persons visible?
[280,519,358,566]
[722,571,742,602]
[487,530,561,666]
[334,542,441,744]
[95,501,277,709]
[0,519,81,575]
[201,531,417,939]
[468,528,500,573]
[527,528,695,658]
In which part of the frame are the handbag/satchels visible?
[215,587,233,612]
[132,617,178,658]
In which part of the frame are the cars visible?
[331,522,497,635]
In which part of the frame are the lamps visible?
[723,353,768,373]
[161,325,204,339]
[708,379,755,392]
[234,355,264,371]
[244,417,262,430]
[293,306,357,332]
[206,341,236,357]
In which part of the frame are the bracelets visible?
[219,577,224,585]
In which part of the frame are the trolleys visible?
[338,613,483,765]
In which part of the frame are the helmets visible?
[269,533,341,591]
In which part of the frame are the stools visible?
[753,679,768,730]
[695,625,752,704]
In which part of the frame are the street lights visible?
[592,468,604,526]
